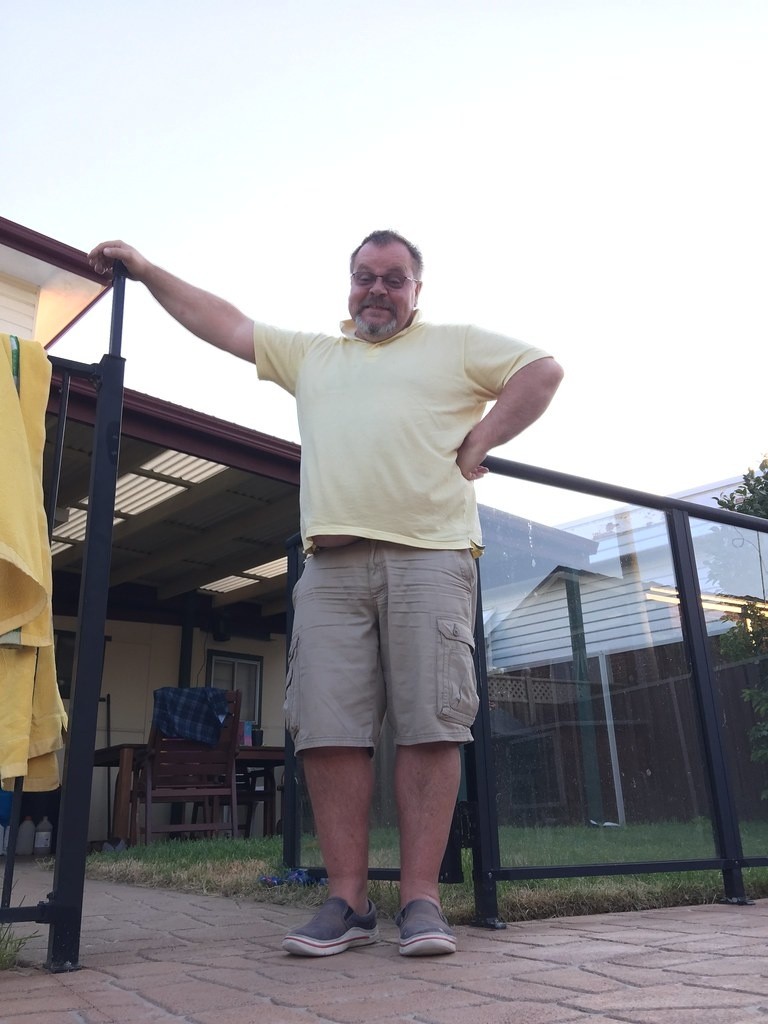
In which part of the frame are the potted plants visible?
[252,724,263,745]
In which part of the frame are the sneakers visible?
[395,899,459,956]
[280,895,381,956]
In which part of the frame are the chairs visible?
[113,687,239,846]
[189,722,274,840]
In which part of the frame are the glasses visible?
[351,271,416,289]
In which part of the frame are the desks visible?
[92,743,284,848]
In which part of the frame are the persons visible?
[86,231,564,957]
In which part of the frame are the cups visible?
[252,730,263,747]
